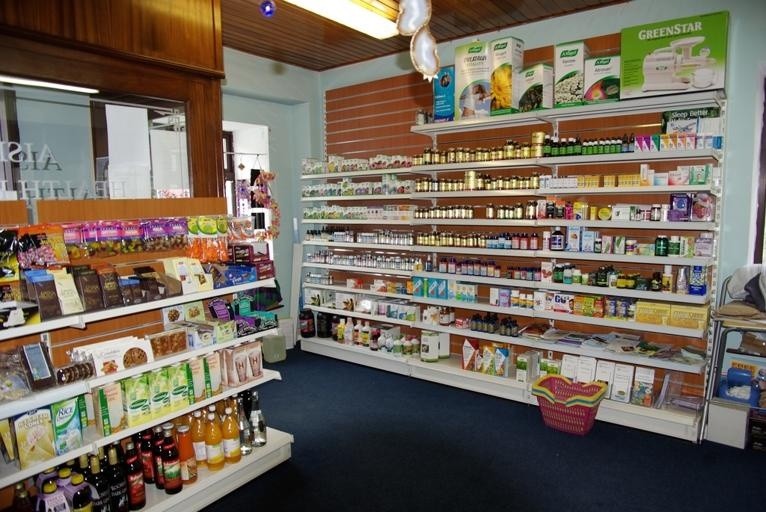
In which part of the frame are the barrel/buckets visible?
[261,327,287,363]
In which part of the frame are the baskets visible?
[531,374,608,436]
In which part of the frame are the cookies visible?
[123,347,148,368]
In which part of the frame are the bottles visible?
[6,389,267,512]
[302,252,689,293]
[649,203,672,222]
[438,305,519,337]
[297,307,420,359]
[413,171,539,191]
[415,110,433,124]
[594,238,603,253]
[411,132,638,167]
[306,225,565,251]
[654,235,668,256]
[413,200,538,220]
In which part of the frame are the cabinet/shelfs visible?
[1,269,293,512]
[293,85,728,447]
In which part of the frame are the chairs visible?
[696,266,765,451]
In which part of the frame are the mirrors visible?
[0,71,192,203]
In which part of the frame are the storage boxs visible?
[554,41,593,109]
[620,10,729,101]
[454,41,490,120]
[518,62,553,113]
[432,65,454,121]
[584,53,620,105]
[489,36,526,116]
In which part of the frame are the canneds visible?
[412,131,545,249]
[617,272,640,289]
